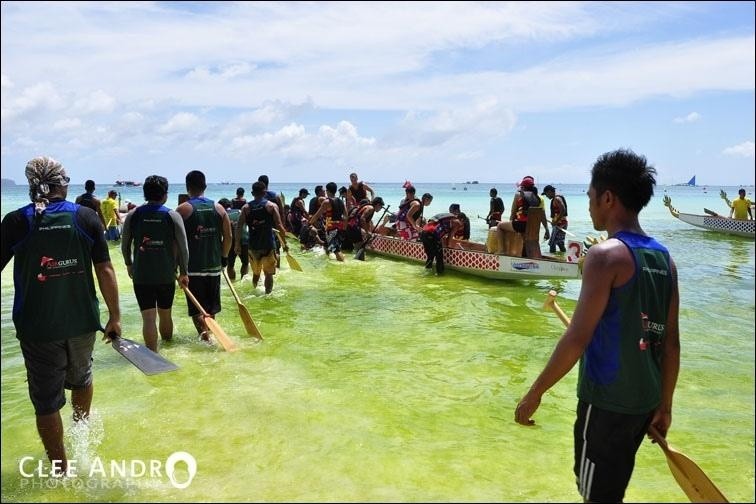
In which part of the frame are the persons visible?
[487,188,504,228]
[121,171,470,356]
[0,156,121,476]
[514,149,680,503]
[494,176,550,255]
[101,190,120,242]
[75,180,105,226]
[541,185,568,252]
[728,189,752,220]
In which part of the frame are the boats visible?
[279,191,606,282]
[662,195,755,239]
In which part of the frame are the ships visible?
[112,180,141,188]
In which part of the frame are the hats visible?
[299,188,310,194]
[541,185,554,195]
[518,178,534,186]
[338,186,347,198]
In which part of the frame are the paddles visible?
[98,327,181,374]
[543,290,731,504]
[357,207,389,260]
[224,268,264,341]
[275,231,300,270]
[175,275,235,352]
